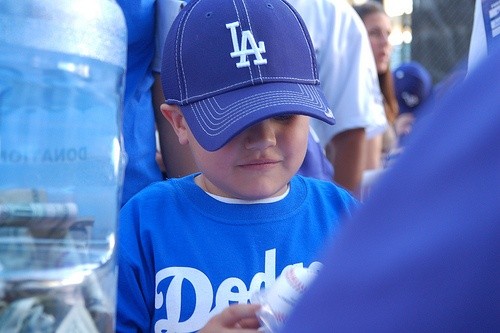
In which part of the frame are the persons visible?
[117,0,500,333]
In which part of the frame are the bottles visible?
[0,0,128,333]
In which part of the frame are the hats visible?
[391,61,433,113]
[161,0,337,151]
